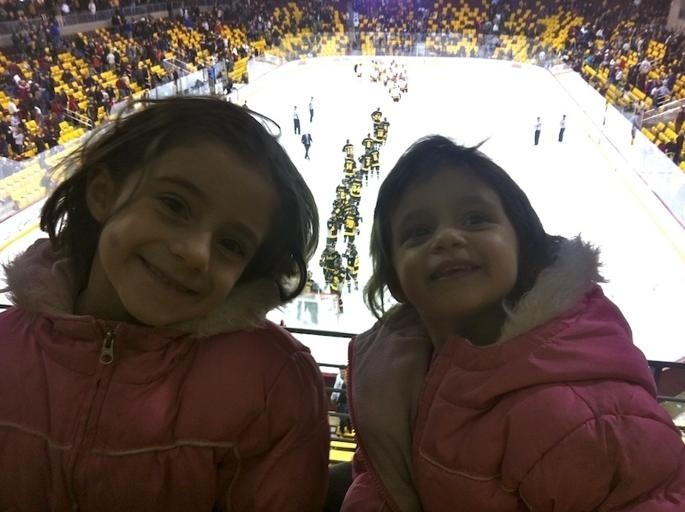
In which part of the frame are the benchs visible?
[327,426,356,463]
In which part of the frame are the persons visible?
[340,138,685,509]
[0,98,330,512]
[331,365,352,435]
[0,1,684,324]
